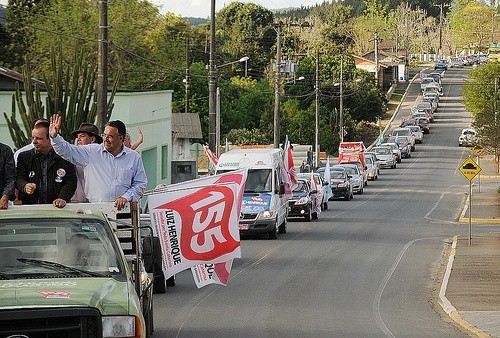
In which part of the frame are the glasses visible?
[100,134,122,140]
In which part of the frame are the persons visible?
[13,118,78,208]
[14,119,144,202]
[49,114,148,254]
[0,141,15,209]
[59,231,108,266]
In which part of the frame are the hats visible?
[71,123,103,143]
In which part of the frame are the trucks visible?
[209,149,292,240]
[0,200,156,338]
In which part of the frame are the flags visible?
[147,167,250,290]
[281,141,299,196]
[311,173,321,216]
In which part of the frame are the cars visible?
[340,152,381,187]
[332,164,365,195]
[371,147,398,169]
[458,129,479,148]
[315,167,354,201]
[284,178,317,222]
[386,53,490,159]
[377,142,403,164]
[295,173,329,212]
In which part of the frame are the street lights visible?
[333,81,344,144]
[273,77,305,149]
[208,55,249,164]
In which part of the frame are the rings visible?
[63,202,65,203]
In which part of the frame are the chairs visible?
[0,249,24,272]
[57,247,70,264]
